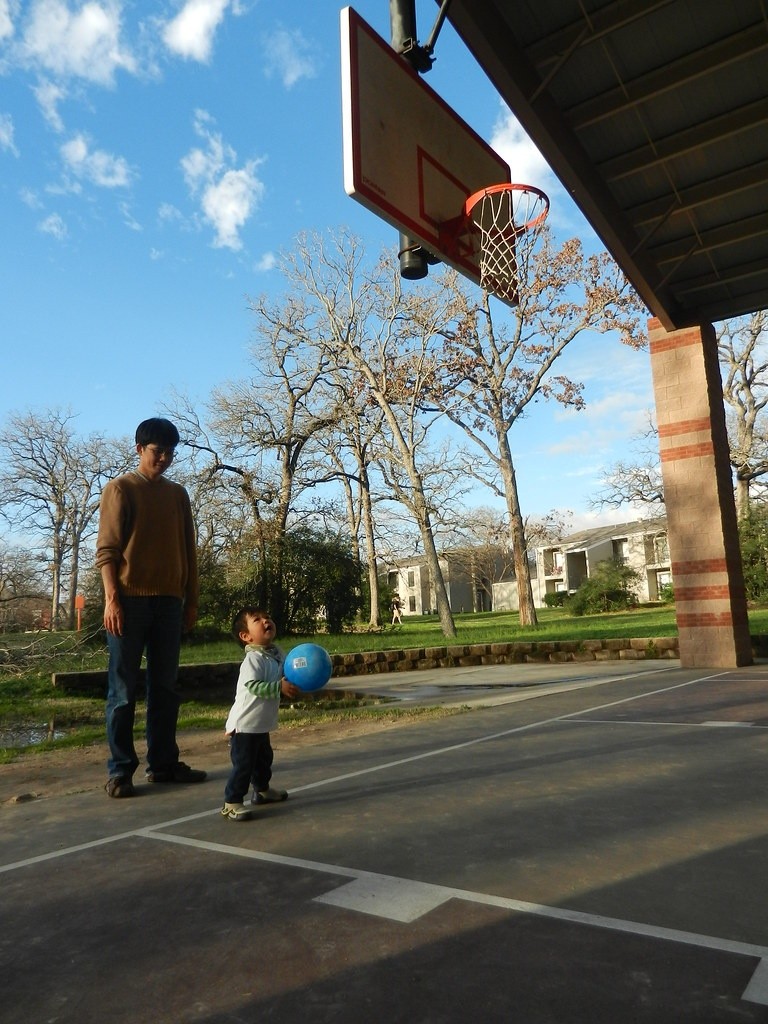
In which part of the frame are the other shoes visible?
[144,761,207,784]
[104,776,135,798]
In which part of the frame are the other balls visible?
[283,642,332,691]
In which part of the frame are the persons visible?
[220,605,300,822]
[389,593,403,624]
[95,418,207,798]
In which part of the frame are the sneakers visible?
[220,802,253,823]
[251,788,288,804]
